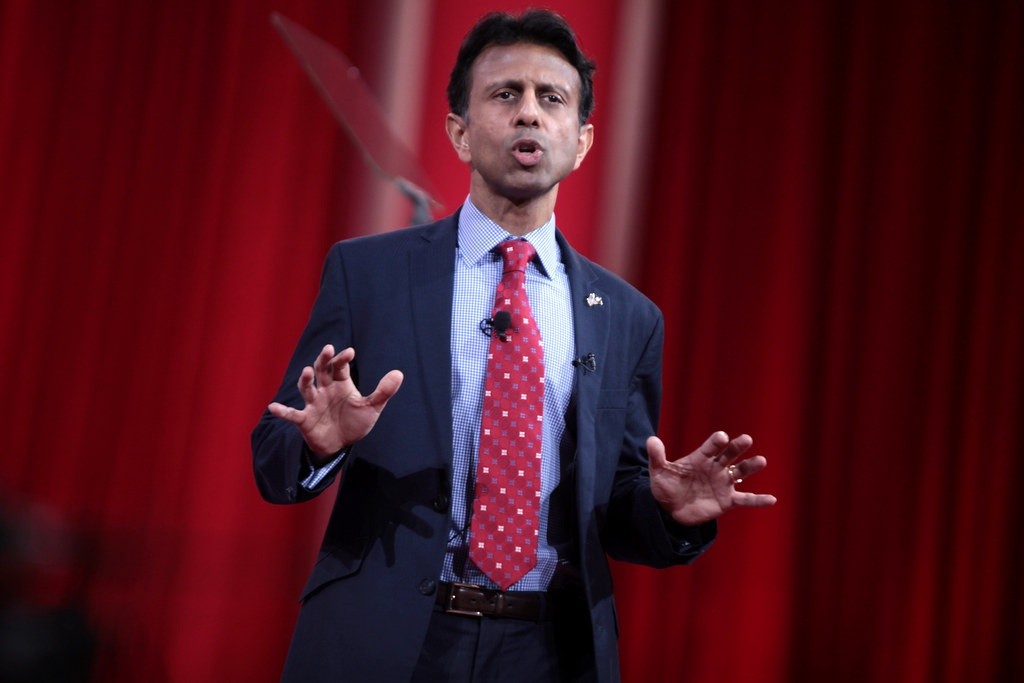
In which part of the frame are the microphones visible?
[494,310,512,342]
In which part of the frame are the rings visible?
[729,465,742,483]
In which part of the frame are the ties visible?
[467,242,546,591]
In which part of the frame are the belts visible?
[437,579,541,622]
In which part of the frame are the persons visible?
[251,10,777,683]
